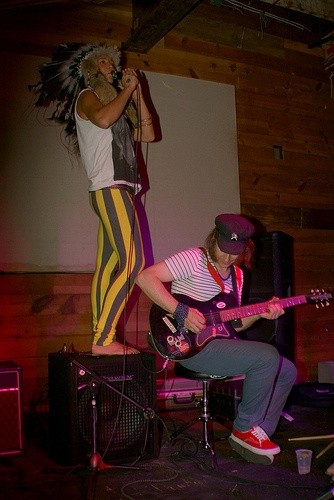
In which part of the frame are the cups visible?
[296,450,312,475]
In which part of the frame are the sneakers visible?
[228,425,281,466]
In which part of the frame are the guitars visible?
[147,289,334,361]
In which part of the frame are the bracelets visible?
[173,303,189,330]
[142,118,153,127]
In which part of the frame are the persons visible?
[74,43,155,354]
[135,213,300,465]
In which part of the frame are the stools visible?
[165,363,232,468]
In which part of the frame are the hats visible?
[215,213,256,255]
[80,45,138,126]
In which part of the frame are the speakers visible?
[240,230,294,360]
[48,351,160,466]
[0,368,24,455]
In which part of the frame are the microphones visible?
[112,71,124,79]
